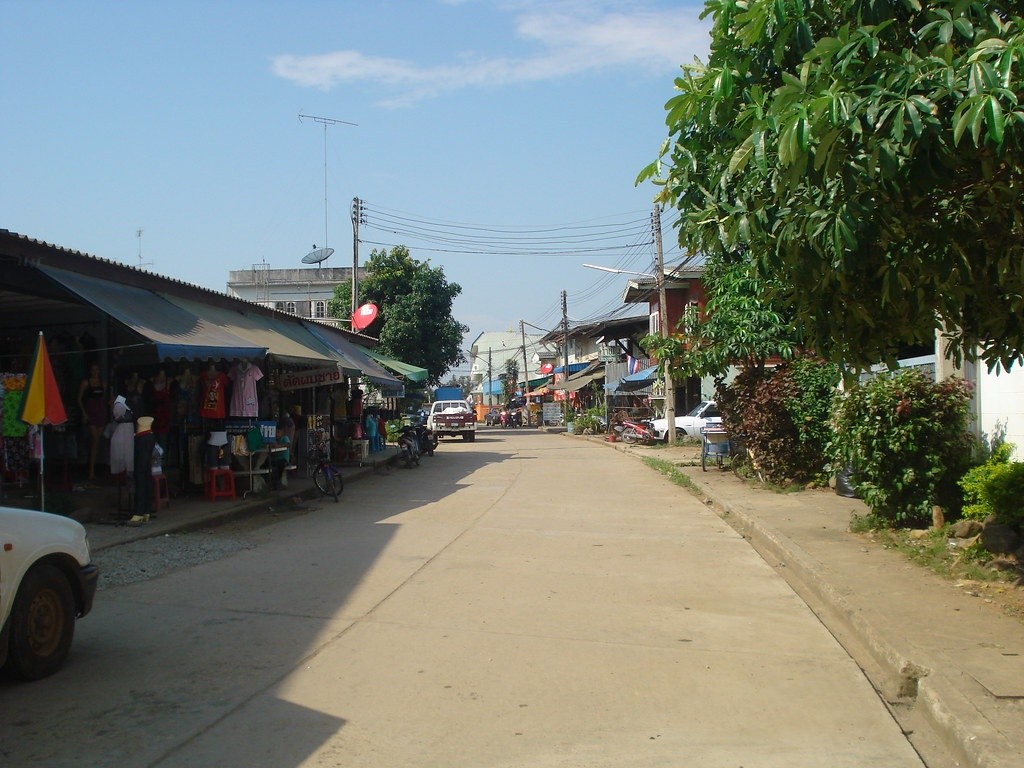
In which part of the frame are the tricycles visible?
[699,422,735,473]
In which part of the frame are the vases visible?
[609,435,616,442]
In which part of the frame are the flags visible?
[628,356,641,375]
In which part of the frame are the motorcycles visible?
[392,421,439,468]
[621,419,657,445]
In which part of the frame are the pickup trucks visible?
[425,400,478,442]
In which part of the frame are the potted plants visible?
[563,412,575,432]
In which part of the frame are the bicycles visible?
[305,436,343,503]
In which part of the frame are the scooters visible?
[499,408,523,429]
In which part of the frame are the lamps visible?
[207,430,230,470]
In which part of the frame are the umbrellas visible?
[17,330,69,513]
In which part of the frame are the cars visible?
[484,406,501,426]
[0,512,92,685]
[649,398,725,443]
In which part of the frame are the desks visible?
[232,446,290,497]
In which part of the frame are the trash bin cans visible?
[567,422,575,432]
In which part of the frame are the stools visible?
[366,435,380,453]
[146,475,170,513]
[204,469,237,504]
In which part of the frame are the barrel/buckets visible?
[609,435,616,442]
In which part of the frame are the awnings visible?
[604,365,658,389]
[351,342,428,384]
[155,290,339,369]
[246,314,362,378]
[27,260,270,368]
[301,320,404,389]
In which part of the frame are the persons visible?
[198,363,228,419]
[318,386,363,420]
[177,368,199,391]
[205,431,232,470]
[124,372,146,412]
[129,416,156,521]
[228,358,265,417]
[366,414,387,453]
[150,370,174,435]
[109,394,135,474]
[77,362,109,483]
[260,428,291,490]
